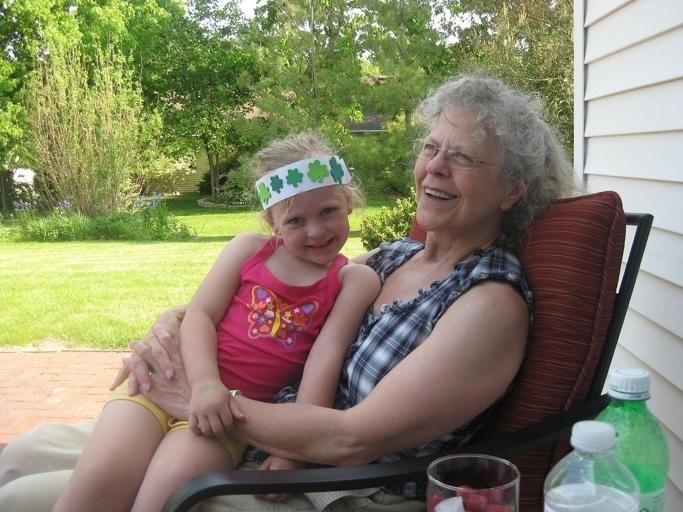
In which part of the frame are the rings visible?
[147,368,152,376]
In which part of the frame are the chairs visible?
[160,204,651,512]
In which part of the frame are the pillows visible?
[413,188,625,512]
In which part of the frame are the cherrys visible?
[426,485,512,512]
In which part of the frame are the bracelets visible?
[229,389,243,398]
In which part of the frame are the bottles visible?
[541,366,671,511]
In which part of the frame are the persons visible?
[1,76,574,512]
[53,132,382,512]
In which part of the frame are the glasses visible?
[413,138,508,171]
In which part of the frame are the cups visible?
[425,454,520,512]
